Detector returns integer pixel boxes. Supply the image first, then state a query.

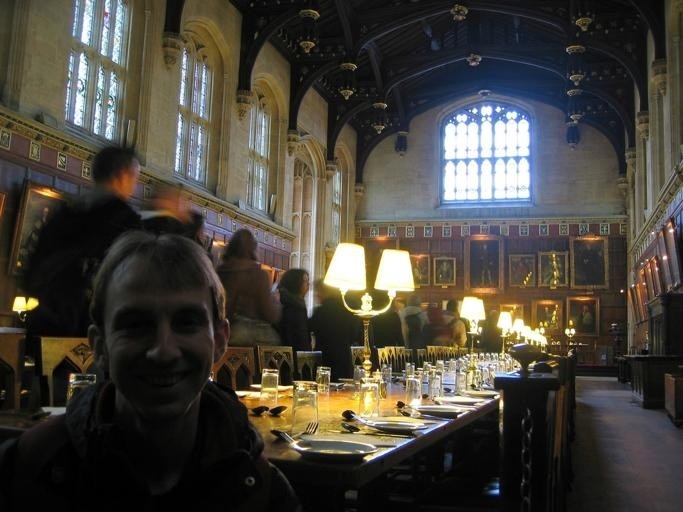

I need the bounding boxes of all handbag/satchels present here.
[227,313,282,347]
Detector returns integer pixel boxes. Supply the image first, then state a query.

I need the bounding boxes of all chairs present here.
[1,325,578,512]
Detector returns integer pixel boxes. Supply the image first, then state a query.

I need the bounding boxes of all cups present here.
[260,352,515,432]
[64,372,97,403]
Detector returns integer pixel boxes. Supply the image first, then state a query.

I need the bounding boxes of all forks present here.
[297,420,320,436]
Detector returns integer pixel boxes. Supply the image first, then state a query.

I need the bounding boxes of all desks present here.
[611,352,682,428]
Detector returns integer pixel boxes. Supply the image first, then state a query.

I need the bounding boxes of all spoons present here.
[0,410,51,420]
[245,404,287,418]
[269,429,289,439]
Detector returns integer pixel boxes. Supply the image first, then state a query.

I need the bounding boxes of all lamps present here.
[296,0,596,159]
[321,241,576,382]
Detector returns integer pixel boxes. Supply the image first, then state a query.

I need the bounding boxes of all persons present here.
[577,241,603,282]
[0,231,304,512]
[512,259,528,284]
[574,302,595,335]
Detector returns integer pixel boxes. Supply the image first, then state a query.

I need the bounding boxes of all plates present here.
[249,383,292,392]
[287,439,378,462]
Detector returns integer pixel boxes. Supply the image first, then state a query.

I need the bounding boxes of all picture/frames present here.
[3,177,80,281]
[408,214,682,340]
